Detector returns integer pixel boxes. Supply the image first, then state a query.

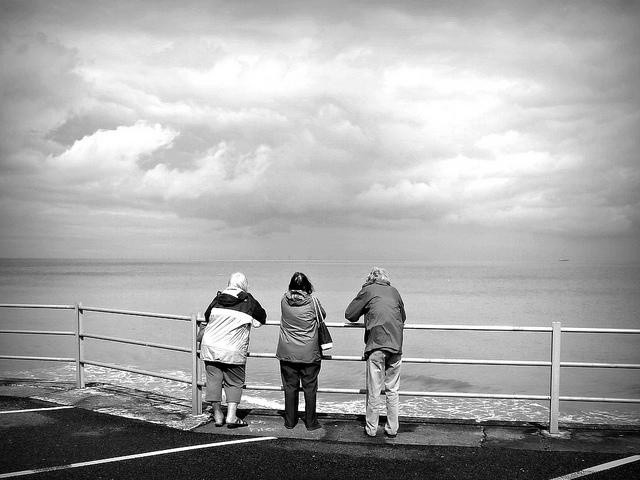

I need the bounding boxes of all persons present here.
[194,273,266,428]
[274,271,326,432]
[343,266,406,437]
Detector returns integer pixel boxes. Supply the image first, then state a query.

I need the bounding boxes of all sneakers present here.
[285,418,297,429]
[306,419,321,431]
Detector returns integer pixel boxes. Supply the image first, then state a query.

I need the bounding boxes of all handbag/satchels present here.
[318,322,333,350]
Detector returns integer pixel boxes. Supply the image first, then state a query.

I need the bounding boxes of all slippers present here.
[215,415,226,427]
[227,417,249,429]
